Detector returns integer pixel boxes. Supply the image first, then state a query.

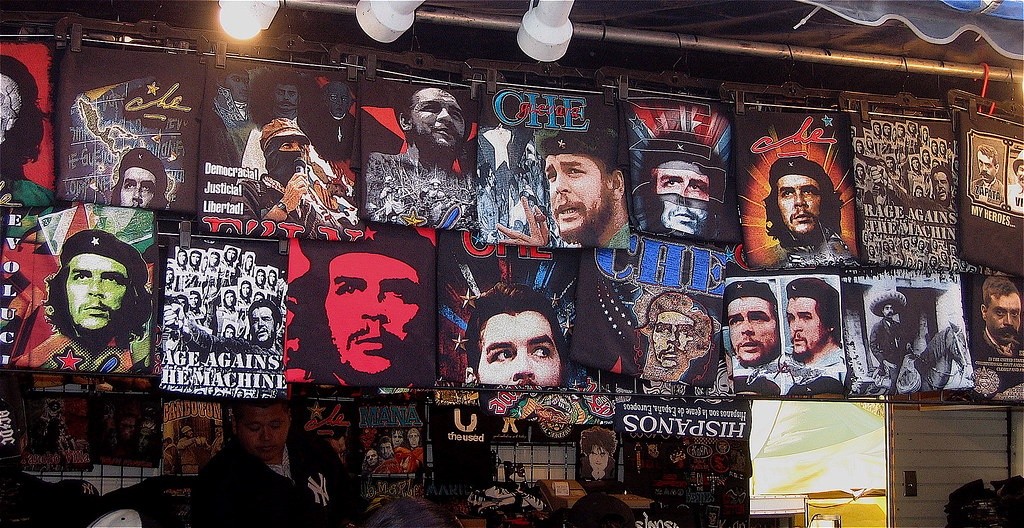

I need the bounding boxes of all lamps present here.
[517,0,574,62]
[356,0,426,42]
[219,0,280,39]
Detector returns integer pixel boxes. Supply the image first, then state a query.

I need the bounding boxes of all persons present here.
[186,397,366,528]
